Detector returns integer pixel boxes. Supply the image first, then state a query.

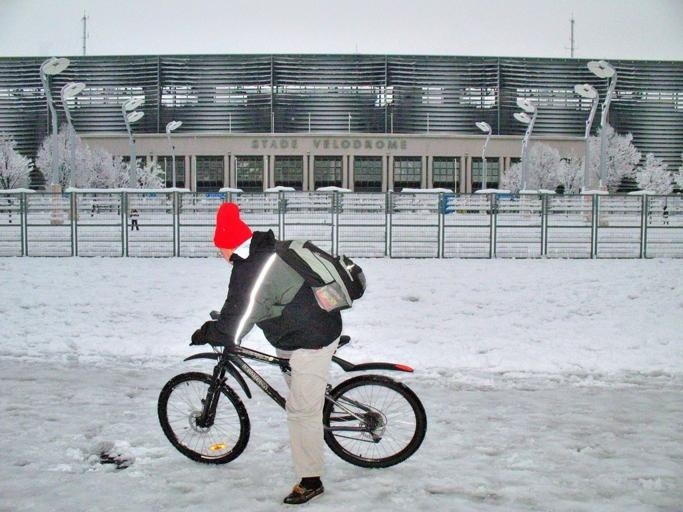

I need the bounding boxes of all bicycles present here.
[157,335,428,469]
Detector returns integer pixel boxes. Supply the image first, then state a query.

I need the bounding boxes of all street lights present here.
[39,55,183,224]
[475,59,618,227]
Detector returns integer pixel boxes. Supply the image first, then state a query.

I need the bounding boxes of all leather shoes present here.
[283,483,325,506]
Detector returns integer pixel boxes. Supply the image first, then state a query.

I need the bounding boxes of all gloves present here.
[191,321,228,345]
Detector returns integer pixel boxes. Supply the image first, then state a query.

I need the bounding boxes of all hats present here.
[214,202,253,249]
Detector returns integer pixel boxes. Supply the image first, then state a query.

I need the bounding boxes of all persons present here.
[196,202,367,504]
[130,209,139,230]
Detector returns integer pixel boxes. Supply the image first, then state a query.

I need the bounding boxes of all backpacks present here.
[254,239,367,314]
